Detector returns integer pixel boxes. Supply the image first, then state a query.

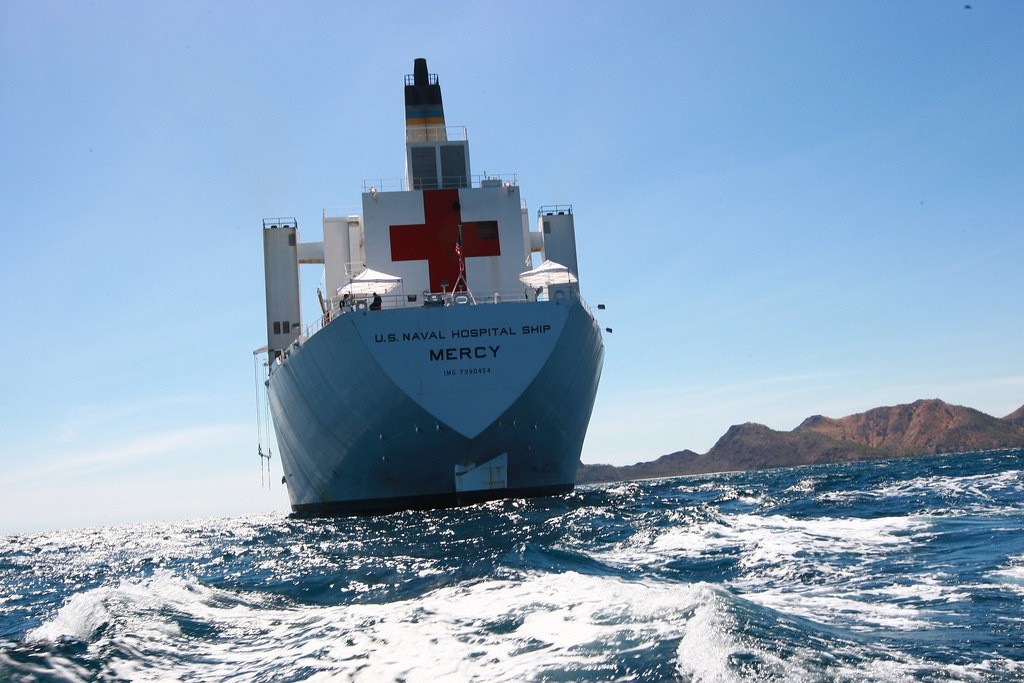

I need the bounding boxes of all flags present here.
[455,233,466,271]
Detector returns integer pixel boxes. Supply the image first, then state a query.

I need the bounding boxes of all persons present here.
[339,294,351,309]
[369,293,382,310]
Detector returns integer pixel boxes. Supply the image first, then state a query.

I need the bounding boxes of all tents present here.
[518,258,578,301]
[337,268,405,314]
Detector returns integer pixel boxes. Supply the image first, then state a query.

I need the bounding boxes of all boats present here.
[261,57,605,514]
